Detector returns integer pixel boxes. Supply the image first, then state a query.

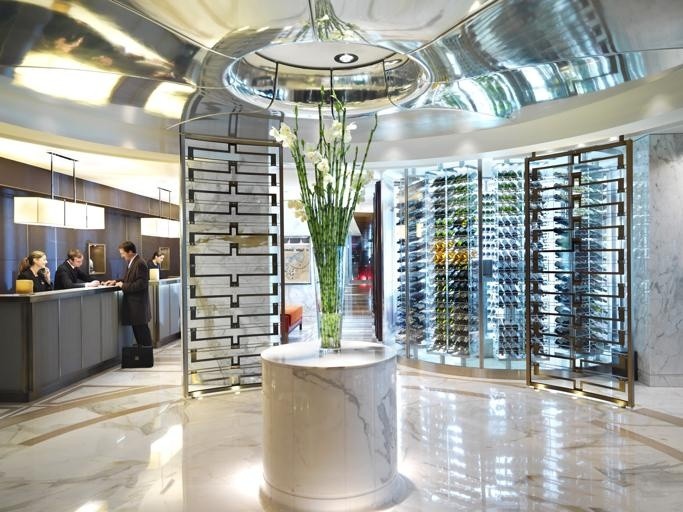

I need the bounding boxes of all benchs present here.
[279,305,303,342]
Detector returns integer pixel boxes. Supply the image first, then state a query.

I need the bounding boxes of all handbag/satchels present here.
[121,342,153,368]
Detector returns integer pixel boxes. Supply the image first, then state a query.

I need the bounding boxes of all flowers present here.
[269,86,378,352]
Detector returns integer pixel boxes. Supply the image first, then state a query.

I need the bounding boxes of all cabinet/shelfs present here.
[527,139,633,409]
[377,157,525,379]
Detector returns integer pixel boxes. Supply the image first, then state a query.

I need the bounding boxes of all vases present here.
[311,245,344,353]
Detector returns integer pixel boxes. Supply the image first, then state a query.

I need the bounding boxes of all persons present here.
[16,251,53,293]
[53,248,104,290]
[102,241,152,346]
[147,251,168,279]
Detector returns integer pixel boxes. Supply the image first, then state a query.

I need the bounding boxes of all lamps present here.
[13,152,105,230]
[140,188,180,239]
